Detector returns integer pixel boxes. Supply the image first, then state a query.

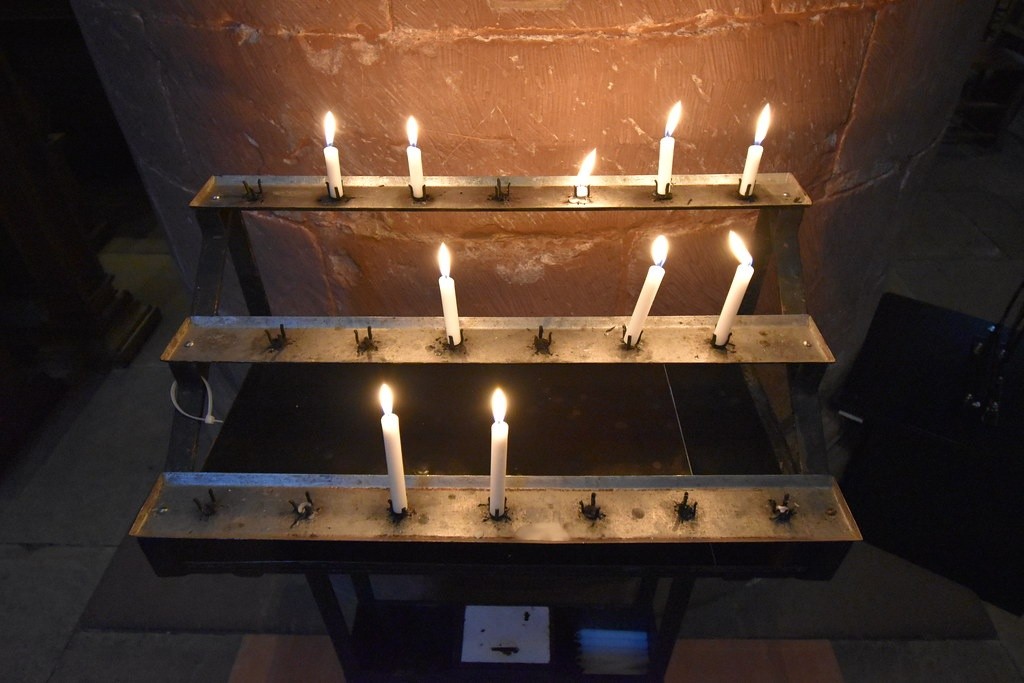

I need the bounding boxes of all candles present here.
[623,235,669,347]
[490,385,510,517]
[437,243,463,346]
[322,111,344,199]
[377,383,410,515]
[739,101,772,197]
[574,147,597,197]
[713,229,754,345]
[657,101,681,194]
[405,116,425,199]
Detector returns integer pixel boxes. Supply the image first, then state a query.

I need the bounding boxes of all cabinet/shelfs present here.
[128,173,865,683]
